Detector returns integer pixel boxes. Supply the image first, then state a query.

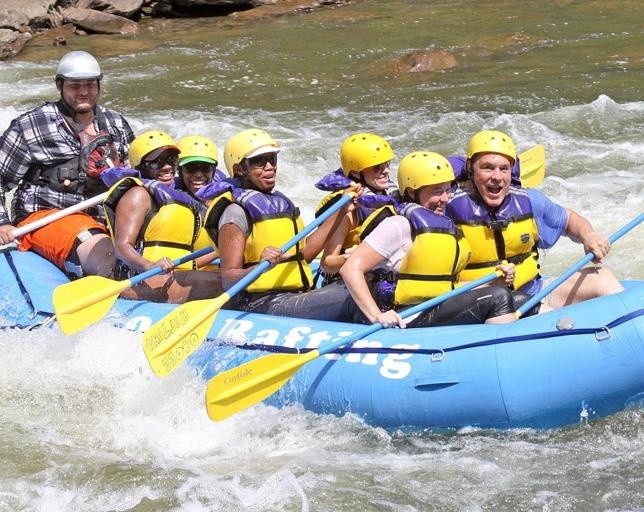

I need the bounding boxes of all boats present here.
[0,224,644,440]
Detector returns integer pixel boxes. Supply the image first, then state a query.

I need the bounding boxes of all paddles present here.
[52,247,214,335]
[518,144,546,187]
[205,259,511,420]
[141,182,357,378]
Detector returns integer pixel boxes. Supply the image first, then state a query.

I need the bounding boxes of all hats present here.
[244,144,282,159]
[179,156,216,166]
[144,146,181,161]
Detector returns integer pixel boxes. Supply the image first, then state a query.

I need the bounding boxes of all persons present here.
[444,130,624,314]
[201,128,377,325]
[101,130,232,309]
[339,151,517,331]
[178,134,226,213]
[0,49,136,279]
[314,133,399,286]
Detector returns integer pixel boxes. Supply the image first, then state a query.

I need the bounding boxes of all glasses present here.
[185,165,213,174]
[142,155,178,171]
[250,156,279,169]
[371,160,391,177]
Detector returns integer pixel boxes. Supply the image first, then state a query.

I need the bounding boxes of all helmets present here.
[129,131,180,169]
[341,133,393,179]
[175,136,218,166]
[54,50,103,81]
[398,152,457,196]
[224,128,277,177]
[465,131,517,167]
